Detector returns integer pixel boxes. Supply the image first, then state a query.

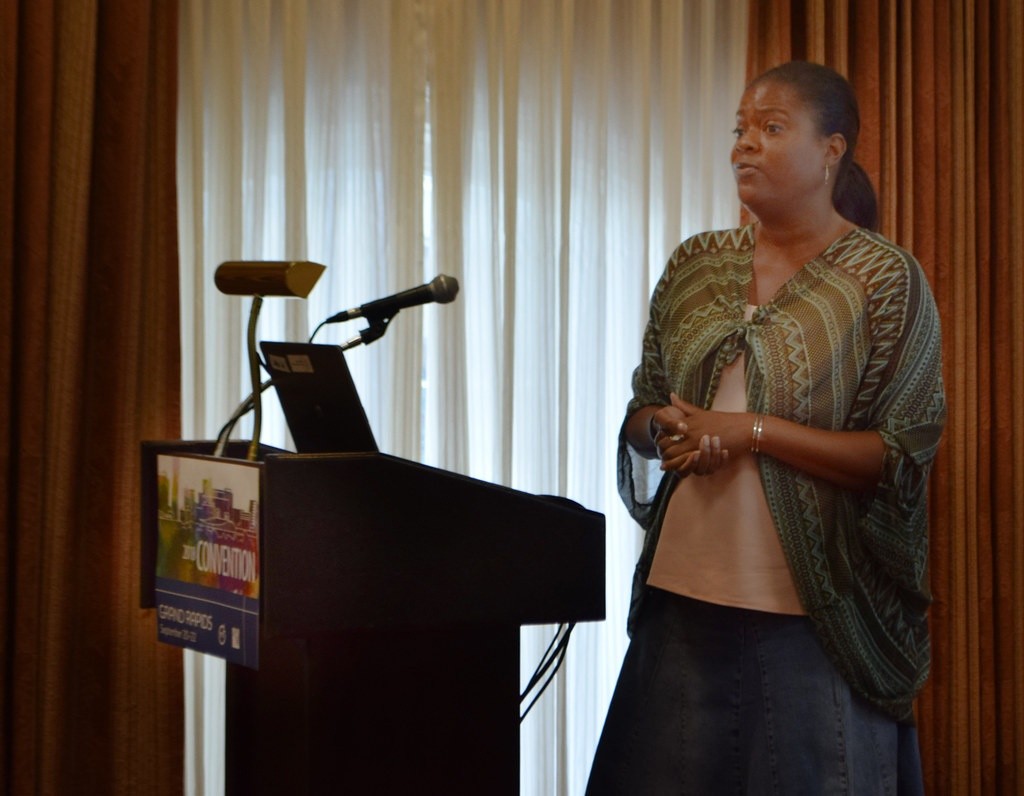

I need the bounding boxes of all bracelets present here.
[756,415,764,452]
[751,415,759,452]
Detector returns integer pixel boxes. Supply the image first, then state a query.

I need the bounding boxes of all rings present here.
[670,432,684,441]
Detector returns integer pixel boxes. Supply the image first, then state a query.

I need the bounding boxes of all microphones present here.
[326,275,459,323]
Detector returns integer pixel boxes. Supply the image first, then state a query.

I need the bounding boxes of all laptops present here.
[259,341,378,454]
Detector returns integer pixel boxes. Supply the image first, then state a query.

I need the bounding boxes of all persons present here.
[584,59,947,796]
